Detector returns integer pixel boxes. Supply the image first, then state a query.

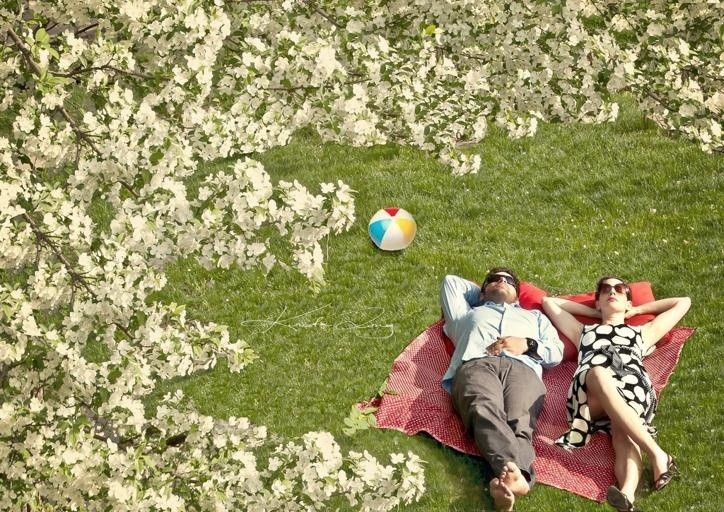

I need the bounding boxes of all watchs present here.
[524,336,538,355]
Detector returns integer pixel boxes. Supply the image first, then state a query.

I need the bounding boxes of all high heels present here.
[653,455,681,491]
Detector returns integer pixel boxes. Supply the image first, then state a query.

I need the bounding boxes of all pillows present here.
[554,283,673,361]
[445,282,546,356]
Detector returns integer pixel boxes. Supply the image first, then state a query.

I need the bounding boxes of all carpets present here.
[362,313,694,505]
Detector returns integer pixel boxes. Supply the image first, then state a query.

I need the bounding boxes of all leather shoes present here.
[606,486,633,512]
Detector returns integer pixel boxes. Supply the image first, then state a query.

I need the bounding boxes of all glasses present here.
[598,283,630,293]
[483,275,515,288]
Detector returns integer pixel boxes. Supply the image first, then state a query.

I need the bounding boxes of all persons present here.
[540,275,692,512]
[439,266,564,512]
